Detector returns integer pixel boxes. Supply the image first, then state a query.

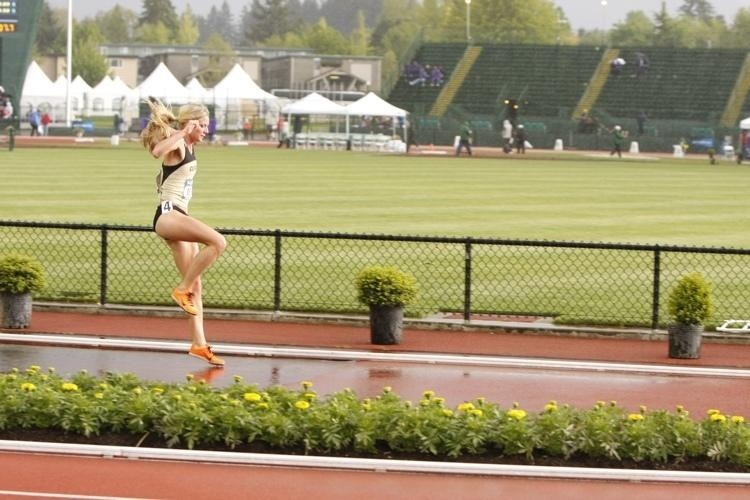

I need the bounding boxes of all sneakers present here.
[189,344,225,365]
[171,288,197,315]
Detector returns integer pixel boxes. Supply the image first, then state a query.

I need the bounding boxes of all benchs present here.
[386,41,749,120]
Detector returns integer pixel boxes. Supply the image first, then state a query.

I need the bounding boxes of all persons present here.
[241,112,298,148]
[404,57,444,89]
[139,96,228,368]
[451,118,526,158]
[678,129,750,168]
[573,52,650,159]
[1,97,54,137]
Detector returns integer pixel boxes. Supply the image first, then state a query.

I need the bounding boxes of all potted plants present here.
[666,267,715,360]
[353,263,419,344]
[0,252,46,332]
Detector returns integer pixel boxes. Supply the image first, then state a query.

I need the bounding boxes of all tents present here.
[279,91,411,150]
[16,59,276,139]
